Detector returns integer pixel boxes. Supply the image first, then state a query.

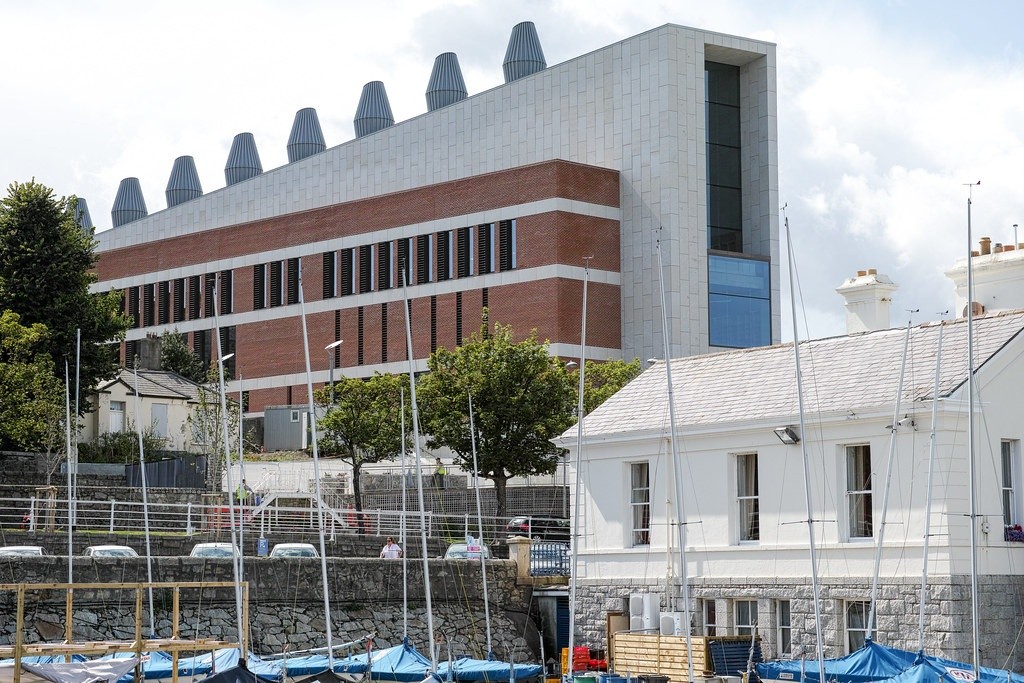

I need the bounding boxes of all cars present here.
[270,542,320,557]
[190,542,240,558]
[0,545,49,557]
[436,541,500,560]
[80,545,139,558]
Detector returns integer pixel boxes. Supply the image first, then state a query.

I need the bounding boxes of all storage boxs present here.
[562,647,607,675]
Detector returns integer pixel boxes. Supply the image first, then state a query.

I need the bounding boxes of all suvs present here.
[506,513,570,546]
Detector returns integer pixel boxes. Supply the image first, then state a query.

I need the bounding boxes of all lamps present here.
[774,426,800,445]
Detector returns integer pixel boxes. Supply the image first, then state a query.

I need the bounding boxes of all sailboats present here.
[0,197,1023,683]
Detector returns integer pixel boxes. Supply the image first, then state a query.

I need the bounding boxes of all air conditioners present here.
[660,611,692,635]
[630,593,660,634]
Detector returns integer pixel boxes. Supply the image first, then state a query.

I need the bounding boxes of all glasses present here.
[386,540,392,542]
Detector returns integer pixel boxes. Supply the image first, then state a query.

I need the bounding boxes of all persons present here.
[237,479,254,520]
[380,537,402,558]
[431,458,445,490]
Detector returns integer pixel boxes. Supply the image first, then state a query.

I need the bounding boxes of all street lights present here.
[324,339,344,434]
